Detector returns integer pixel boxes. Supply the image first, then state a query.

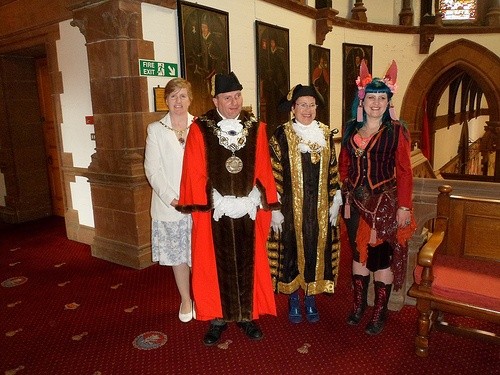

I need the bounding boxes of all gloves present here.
[270,209,284,233]
[328,202,339,226]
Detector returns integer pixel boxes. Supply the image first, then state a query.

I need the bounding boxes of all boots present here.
[364,279,391,335]
[289,290,302,323]
[304,295,319,323]
[348,274,370,325]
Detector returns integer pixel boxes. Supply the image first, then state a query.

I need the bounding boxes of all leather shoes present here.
[205,321,228,343]
[237,320,262,339]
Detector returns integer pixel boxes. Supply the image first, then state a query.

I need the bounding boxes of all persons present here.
[179,71,280,345]
[265,83,344,324]
[143,78,199,322]
[338,59,417,335]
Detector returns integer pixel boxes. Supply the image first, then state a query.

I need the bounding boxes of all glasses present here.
[294,102,319,109]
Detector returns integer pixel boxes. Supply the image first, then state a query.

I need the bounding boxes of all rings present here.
[405,220,411,225]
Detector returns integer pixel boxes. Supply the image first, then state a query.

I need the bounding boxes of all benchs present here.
[407,184,500,358]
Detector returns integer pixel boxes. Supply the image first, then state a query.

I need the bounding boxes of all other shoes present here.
[193,301,197,319]
[178,299,192,322]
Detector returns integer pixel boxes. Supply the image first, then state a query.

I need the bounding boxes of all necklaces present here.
[172,129,188,143]
[354,127,374,157]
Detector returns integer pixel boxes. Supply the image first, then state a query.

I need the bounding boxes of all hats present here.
[278,84,325,112]
[210,72,243,97]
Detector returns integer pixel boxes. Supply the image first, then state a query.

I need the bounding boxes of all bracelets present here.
[399,207,410,211]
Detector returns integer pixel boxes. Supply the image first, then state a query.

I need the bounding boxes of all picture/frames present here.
[177,0,373,148]
